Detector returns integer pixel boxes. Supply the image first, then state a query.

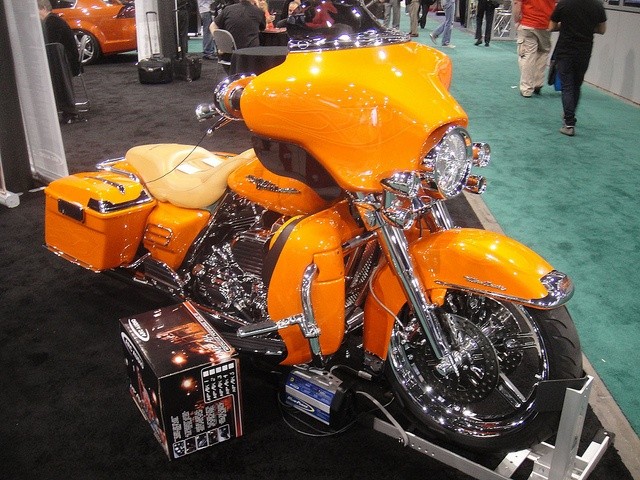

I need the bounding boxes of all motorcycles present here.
[40,0,583,457]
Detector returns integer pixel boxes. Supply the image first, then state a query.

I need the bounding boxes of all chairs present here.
[72,34,89,116]
[213,29,236,85]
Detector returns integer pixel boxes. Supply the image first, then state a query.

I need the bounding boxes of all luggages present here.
[171,10,202,82]
[138,11,169,84]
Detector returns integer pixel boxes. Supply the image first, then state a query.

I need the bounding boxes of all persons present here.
[274,0,302,29]
[255,0,274,29]
[429,0,457,47]
[511,0,562,98]
[548,0,608,136]
[378,0,402,30]
[36,0,79,125]
[404,0,421,38]
[474,0,499,47]
[197,0,220,61]
[459,0,468,28]
[208,0,265,76]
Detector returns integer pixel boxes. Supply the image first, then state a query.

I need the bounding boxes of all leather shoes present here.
[203,54,218,60]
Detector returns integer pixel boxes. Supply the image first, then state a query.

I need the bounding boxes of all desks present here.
[496,12,513,37]
[230,46,287,74]
[258,29,289,40]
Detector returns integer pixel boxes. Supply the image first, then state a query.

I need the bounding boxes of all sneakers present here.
[474,39,482,45]
[442,43,456,48]
[561,113,566,124]
[429,33,437,45]
[408,33,419,37]
[560,126,574,136]
[520,90,531,97]
[533,86,544,94]
[485,42,490,47]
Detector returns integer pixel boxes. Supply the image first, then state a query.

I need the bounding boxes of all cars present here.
[37,0,137,67]
